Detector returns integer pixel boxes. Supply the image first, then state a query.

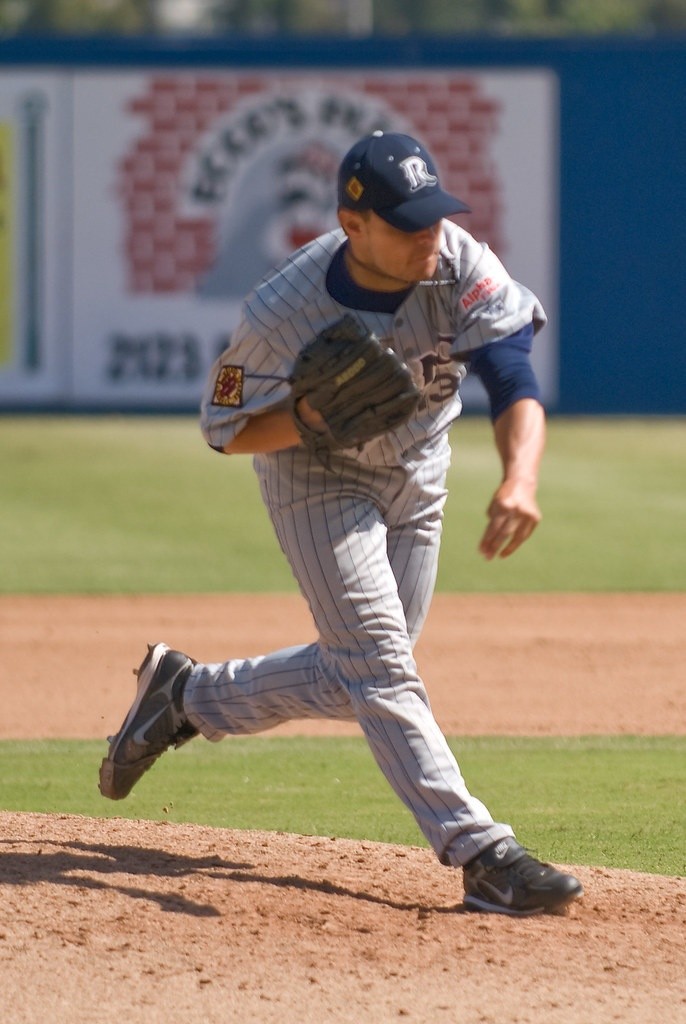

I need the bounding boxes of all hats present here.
[337,132,474,231]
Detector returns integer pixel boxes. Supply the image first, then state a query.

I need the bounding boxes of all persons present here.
[97,128,585,919]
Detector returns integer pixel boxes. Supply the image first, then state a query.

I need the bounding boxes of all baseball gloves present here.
[261,309,426,476]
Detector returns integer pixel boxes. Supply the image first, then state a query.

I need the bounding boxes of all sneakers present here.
[98,642,202,800]
[463,835,584,914]
